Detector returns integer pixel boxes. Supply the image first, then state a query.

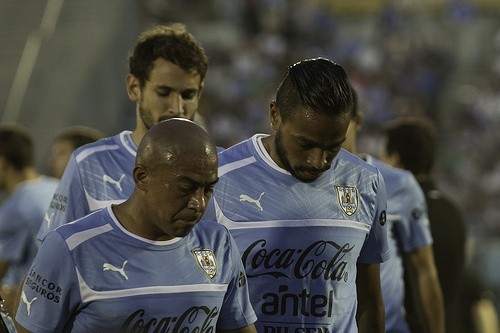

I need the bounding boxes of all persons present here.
[13,118,259,333]
[36,22,228,245]
[201,57,392,333]
[0,87,500,333]
